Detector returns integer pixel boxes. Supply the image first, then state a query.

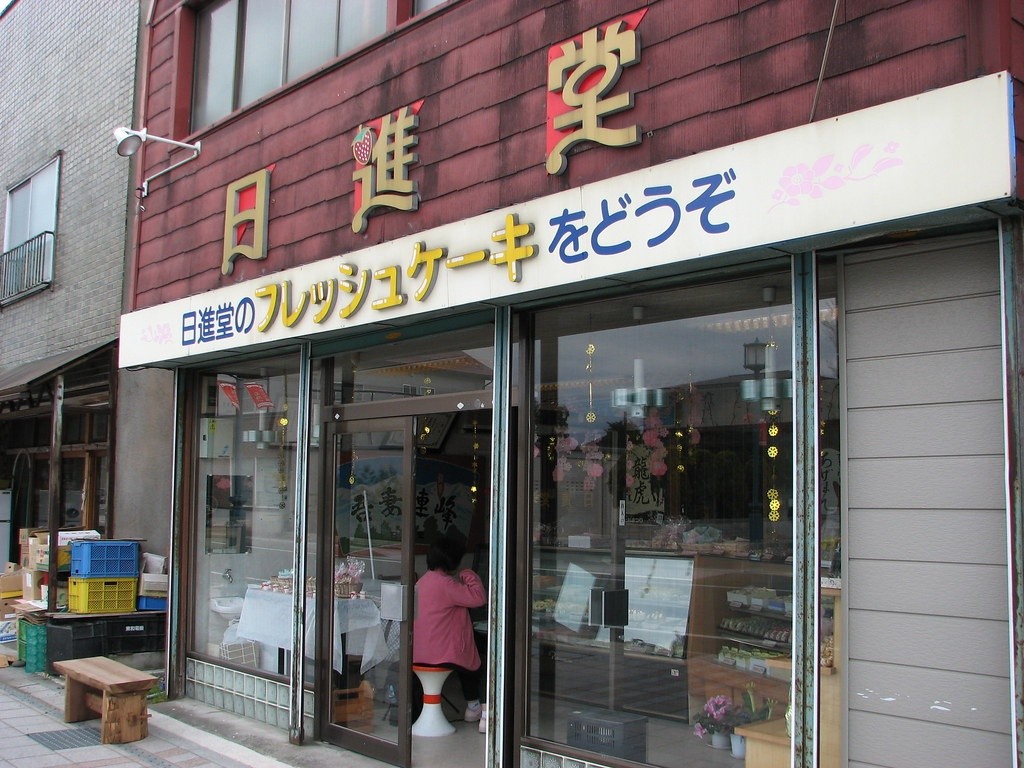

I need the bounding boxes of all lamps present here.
[610,306,670,419]
[742,287,792,411]
[242,367,280,449]
[112,126,202,157]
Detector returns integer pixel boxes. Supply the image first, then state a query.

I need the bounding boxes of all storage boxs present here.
[0,529,168,664]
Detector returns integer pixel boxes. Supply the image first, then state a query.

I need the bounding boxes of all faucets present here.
[223,568,233,583]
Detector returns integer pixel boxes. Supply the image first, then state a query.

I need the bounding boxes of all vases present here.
[711,730,747,757]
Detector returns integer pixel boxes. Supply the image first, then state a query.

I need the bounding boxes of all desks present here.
[246,579,459,721]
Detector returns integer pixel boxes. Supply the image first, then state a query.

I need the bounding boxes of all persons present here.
[413,537,490,733]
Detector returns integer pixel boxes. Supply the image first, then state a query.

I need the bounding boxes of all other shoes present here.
[465,706,481,722]
[479,718,486,732]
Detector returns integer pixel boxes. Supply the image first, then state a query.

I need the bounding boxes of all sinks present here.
[210,597,244,619]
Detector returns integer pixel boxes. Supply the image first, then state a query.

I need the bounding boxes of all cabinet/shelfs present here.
[529,547,695,726]
[694,554,842,768]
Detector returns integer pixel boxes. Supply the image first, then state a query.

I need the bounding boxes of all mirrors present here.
[205,474,254,555]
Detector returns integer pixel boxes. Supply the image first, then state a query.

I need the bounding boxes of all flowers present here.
[692,682,778,739]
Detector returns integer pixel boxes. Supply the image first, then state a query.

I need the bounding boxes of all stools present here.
[412,665,456,737]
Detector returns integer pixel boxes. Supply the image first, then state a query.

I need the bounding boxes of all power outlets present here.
[142,181,149,198]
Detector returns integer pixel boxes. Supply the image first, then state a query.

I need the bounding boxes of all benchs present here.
[52,656,158,744]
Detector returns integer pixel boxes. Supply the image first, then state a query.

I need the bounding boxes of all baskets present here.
[678,540,789,563]
[305,576,316,591]
[334,582,363,598]
[270,576,293,589]
